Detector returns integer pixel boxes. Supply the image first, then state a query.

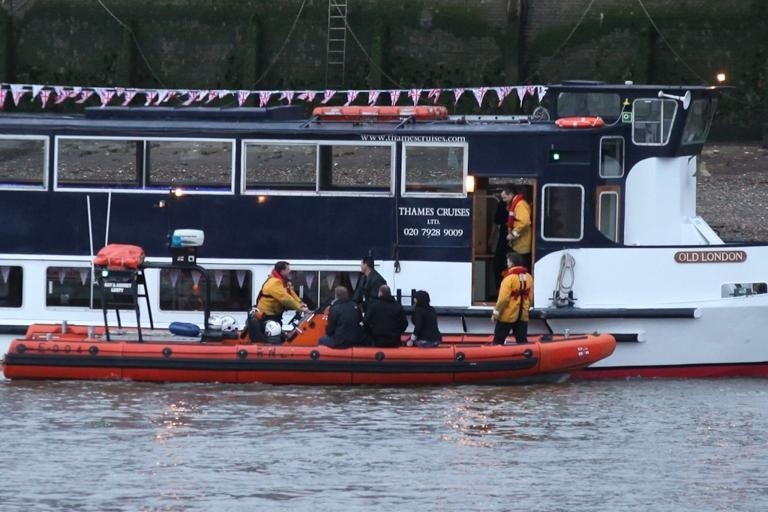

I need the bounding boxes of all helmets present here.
[220,317,240,332]
[208,316,222,332]
[266,321,283,338]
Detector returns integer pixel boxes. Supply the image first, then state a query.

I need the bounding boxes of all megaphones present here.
[658,88,691,110]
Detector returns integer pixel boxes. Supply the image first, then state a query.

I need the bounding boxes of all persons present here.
[352,256,388,314]
[317,285,363,350]
[490,250,534,348]
[364,284,409,347]
[493,182,518,301]
[500,184,532,274]
[406,290,443,349]
[255,260,309,327]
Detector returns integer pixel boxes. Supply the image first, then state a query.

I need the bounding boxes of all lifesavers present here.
[554,115,605,128]
[170,322,201,337]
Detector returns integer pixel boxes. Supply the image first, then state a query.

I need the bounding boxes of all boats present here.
[1,83,767,381]
[3,325,617,382]
